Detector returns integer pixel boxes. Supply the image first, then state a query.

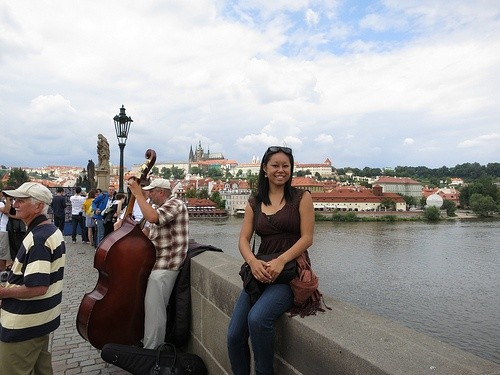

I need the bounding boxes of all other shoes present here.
[5,263,13,271]
[82,241,85,243]
[1,273,8,282]
[90,241,95,246]
[72,241,75,243]
[64,241,67,244]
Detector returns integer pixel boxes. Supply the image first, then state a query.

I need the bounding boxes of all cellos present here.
[76,149,156,350]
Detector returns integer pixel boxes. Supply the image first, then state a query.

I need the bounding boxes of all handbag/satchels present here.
[238,251,297,298]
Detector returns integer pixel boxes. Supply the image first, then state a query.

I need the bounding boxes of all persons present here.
[0,186,27,272]
[226,146,316,375]
[0,180,66,375]
[51,187,66,233]
[69,176,150,251]
[126,177,190,351]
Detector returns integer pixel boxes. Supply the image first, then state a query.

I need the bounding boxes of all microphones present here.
[0,272,8,288]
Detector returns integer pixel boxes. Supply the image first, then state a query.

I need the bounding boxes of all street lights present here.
[113,105,134,194]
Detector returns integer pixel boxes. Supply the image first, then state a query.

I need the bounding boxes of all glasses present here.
[266,146,292,155]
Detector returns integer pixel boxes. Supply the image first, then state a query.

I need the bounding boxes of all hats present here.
[142,178,171,191]
[2,182,54,206]
